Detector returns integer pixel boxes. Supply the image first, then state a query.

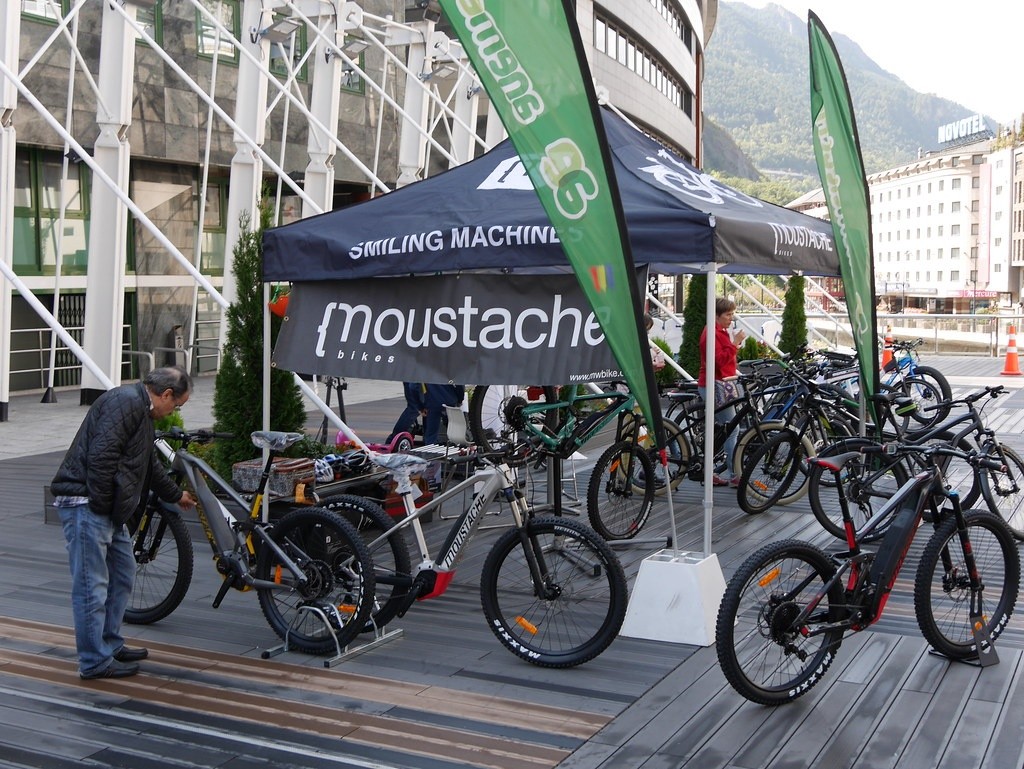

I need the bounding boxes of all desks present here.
[220,466,423,521]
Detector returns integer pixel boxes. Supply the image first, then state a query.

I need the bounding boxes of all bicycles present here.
[123,337,1024,708]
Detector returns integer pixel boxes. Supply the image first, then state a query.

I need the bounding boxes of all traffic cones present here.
[878,324,898,374]
[999,325,1022,375]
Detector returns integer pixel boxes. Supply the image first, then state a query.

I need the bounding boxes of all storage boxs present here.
[383,492,435,524]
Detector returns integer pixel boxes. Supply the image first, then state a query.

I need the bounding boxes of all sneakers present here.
[113,645,148,662]
[729,475,754,488]
[701,473,729,487]
[80,658,139,679]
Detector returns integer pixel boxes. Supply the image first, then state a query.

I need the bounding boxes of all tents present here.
[252,89,867,560]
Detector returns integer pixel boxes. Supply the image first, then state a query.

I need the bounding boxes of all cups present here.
[655,352,664,365]
[733,329,741,345]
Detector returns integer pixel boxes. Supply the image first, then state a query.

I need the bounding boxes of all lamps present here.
[325,40,372,64]
[250,18,302,43]
[418,65,457,86]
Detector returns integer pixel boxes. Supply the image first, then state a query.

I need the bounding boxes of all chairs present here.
[525,454,578,507]
[439,403,524,531]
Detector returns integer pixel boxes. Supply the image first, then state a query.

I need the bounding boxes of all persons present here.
[698,297,746,488]
[47,364,196,679]
[641,311,666,372]
[385,383,464,443]
